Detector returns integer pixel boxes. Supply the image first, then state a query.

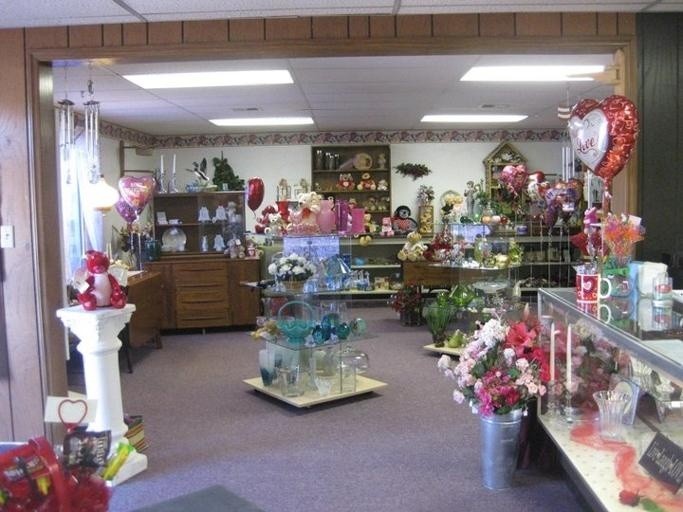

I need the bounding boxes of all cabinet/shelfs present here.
[404,261,504,324]
[256,237,404,308]
[244,231,389,411]
[120,272,162,372]
[311,142,392,233]
[149,191,247,258]
[483,141,529,202]
[487,233,578,293]
[140,258,260,336]
[537,288,683,511]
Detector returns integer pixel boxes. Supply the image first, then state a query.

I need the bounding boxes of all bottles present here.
[314,149,340,170]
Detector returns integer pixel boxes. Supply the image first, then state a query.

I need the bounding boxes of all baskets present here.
[276,301,316,345]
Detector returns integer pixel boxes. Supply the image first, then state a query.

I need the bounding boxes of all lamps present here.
[87,173,119,217]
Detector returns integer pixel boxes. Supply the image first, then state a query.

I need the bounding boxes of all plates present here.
[352,153,373,171]
[161,228,187,246]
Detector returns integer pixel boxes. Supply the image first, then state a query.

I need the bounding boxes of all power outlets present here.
[1,227,15,249]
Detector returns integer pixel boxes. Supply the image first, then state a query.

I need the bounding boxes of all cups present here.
[576,301,612,323]
[651,278,675,308]
[652,307,672,331]
[223,183,228,191]
[247,248,258,257]
[577,274,612,303]
[592,390,631,437]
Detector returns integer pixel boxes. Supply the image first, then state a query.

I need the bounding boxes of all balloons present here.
[501,163,584,232]
[244,176,264,210]
[114,196,144,225]
[569,95,640,181]
[118,174,157,215]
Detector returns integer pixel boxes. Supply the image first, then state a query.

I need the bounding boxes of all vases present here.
[282,281,306,291]
[480,414,520,491]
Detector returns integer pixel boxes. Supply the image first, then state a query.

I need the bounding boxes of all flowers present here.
[437,319,550,417]
[553,321,615,412]
[268,253,317,281]
[441,192,463,220]
[392,286,421,315]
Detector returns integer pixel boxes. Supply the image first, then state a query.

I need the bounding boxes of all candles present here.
[160,154,164,173]
[172,154,177,172]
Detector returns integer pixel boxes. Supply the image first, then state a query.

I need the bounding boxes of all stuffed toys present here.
[254,171,429,261]
